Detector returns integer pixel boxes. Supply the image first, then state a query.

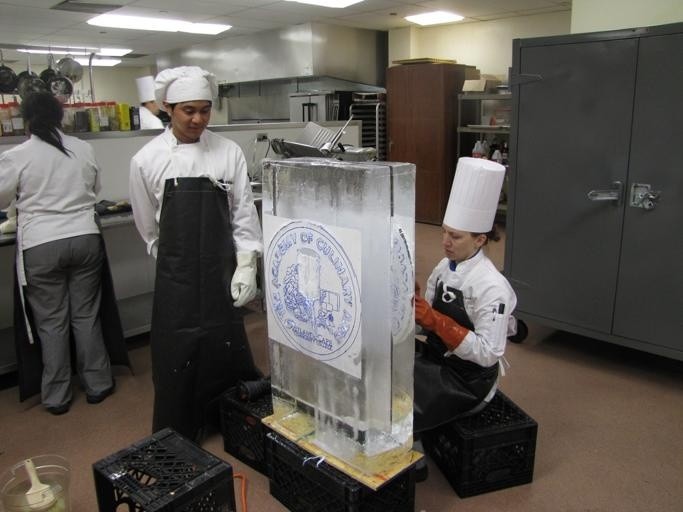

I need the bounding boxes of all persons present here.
[136,76,164,130]
[414,157,517,485]
[129,65,263,445]
[0,91,135,415]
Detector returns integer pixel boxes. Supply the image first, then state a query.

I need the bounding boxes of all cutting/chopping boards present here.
[393,59,457,65]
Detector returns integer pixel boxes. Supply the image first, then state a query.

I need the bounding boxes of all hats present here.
[154,66,218,116]
[136,76,156,103]
[443,157,505,233]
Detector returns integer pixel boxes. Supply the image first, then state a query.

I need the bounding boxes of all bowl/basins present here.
[329,151,378,163]
[2,455,73,507]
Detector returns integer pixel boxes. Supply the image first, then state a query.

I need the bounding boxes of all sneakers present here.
[415,465,428,482]
[86,376,115,404]
[48,402,69,414]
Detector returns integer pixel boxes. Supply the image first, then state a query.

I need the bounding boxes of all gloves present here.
[415,281,420,297]
[231,252,257,308]
[151,239,160,259]
[415,294,469,352]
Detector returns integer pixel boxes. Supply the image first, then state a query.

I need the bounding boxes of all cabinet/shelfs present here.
[502,23,683,359]
[457,94,511,224]
[387,64,476,226]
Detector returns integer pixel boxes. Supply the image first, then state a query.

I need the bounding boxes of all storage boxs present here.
[92,426,238,512]
[267,430,417,512]
[420,387,540,500]
[221,382,274,477]
[462,80,502,96]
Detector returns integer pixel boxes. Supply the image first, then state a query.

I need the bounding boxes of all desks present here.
[0,182,263,380]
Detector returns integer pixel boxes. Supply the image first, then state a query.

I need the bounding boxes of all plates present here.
[467,126,509,129]
